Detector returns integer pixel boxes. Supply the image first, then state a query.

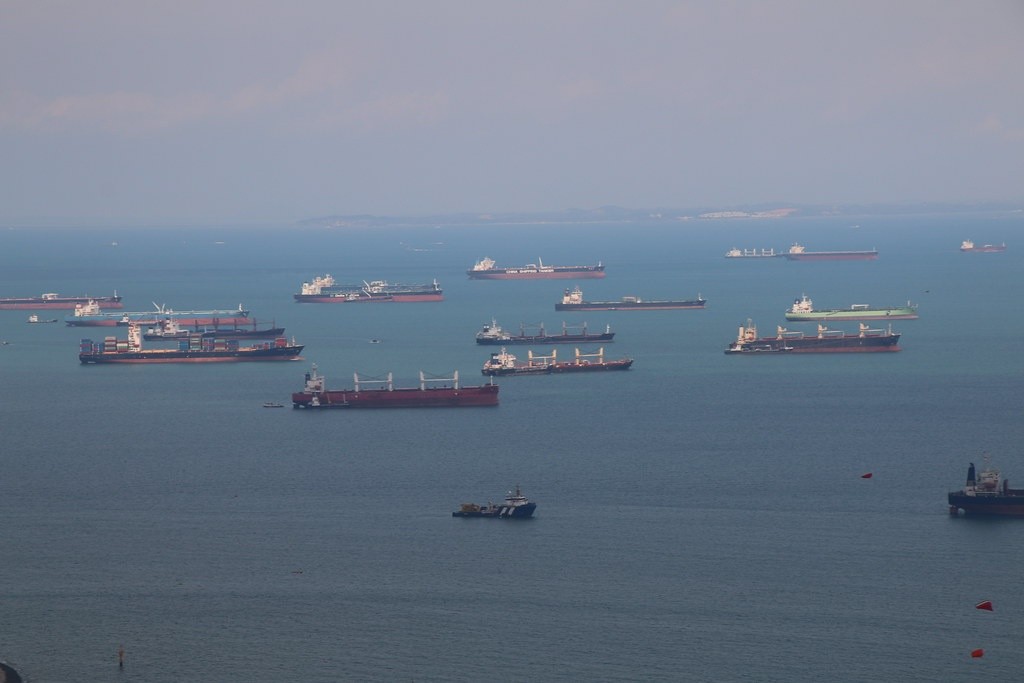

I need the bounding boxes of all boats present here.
[452,484,536,518]
[481,346,634,376]
[64,298,251,328]
[291,363,499,407]
[475,317,615,346]
[785,293,919,320]
[960,238,1008,252]
[947,448,1024,517]
[312,273,440,290]
[143,317,286,342]
[554,285,709,310]
[724,319,902,353]
[725,246,784,258]
[27,314,57,324]
[0,290,124,310]
[464,256,606,280]
[263,401,283,408]
[781,243,879,260]
[79,323,306,362]
[293,281,444,303]
